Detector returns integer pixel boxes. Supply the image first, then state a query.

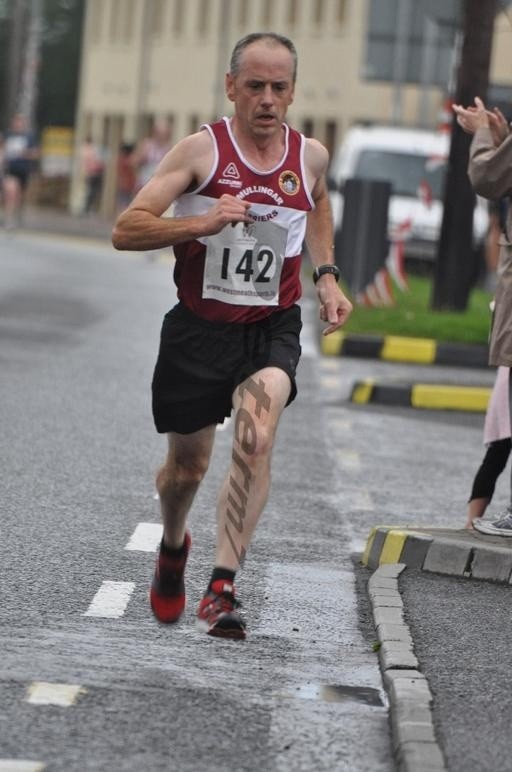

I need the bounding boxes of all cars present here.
[325,119,492,288]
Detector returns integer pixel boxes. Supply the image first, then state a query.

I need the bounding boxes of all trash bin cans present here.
[344,179,388,295]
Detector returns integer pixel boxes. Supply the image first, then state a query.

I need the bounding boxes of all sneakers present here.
[198,579,248,641]
[148,526,191,619]
[471,509,512,536]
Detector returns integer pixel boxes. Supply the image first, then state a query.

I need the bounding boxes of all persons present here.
[80,136,104,213]
[0,114,40,231]
[111,31,353,639]
[462,365,512,530]
[128,117,172,196]
[450,95,512,536]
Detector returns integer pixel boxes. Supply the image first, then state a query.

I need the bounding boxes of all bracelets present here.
[313,264,340,286]
